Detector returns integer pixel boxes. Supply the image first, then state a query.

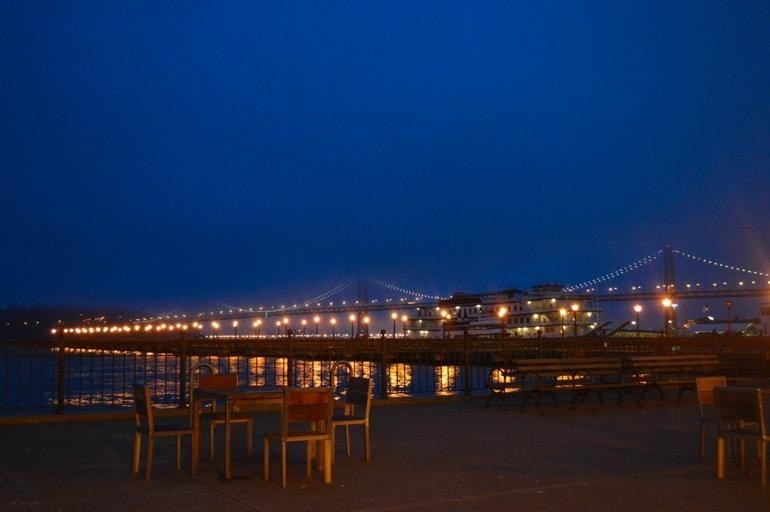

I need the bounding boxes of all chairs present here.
[130,359,375,486]
[692,372,770,486]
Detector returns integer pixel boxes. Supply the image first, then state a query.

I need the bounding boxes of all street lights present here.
[724,300,732,336]
[634,305,642,338]
[440,310,451,339]
[571,303,580,338]
[663,299,670,334]
[498,306,507,337]
[559,308,566,338]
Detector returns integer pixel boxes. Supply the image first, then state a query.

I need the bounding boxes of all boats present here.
[404,284,606,337]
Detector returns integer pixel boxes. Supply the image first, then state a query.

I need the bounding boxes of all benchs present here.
[485,350,730,412]
